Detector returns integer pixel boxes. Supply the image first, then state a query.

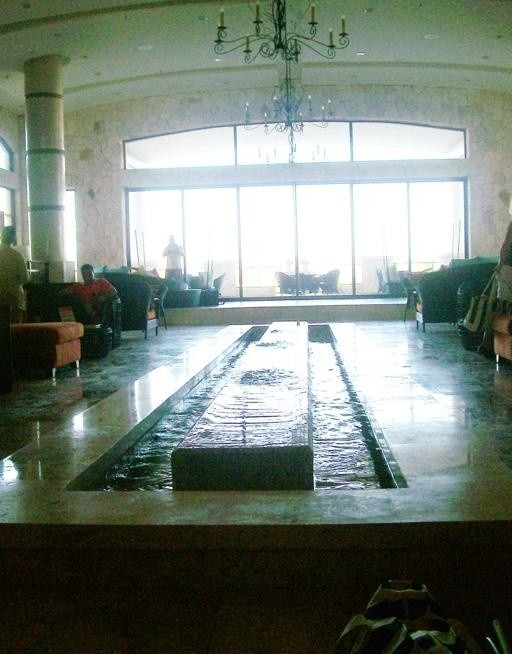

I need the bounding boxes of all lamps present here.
[209,0,351,63]
[238,66,338,134]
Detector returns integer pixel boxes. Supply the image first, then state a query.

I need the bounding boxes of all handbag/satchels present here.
[462,270,497,335]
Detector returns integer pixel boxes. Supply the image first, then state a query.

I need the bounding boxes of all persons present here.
[0,226,29,323]
[478,221,512,360]
[65,264,117,323]
[163,235,184,279]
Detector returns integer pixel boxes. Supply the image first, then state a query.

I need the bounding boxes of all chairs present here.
[375,249,512,374]
[274,267,340,297]
[0,265,227,388]
